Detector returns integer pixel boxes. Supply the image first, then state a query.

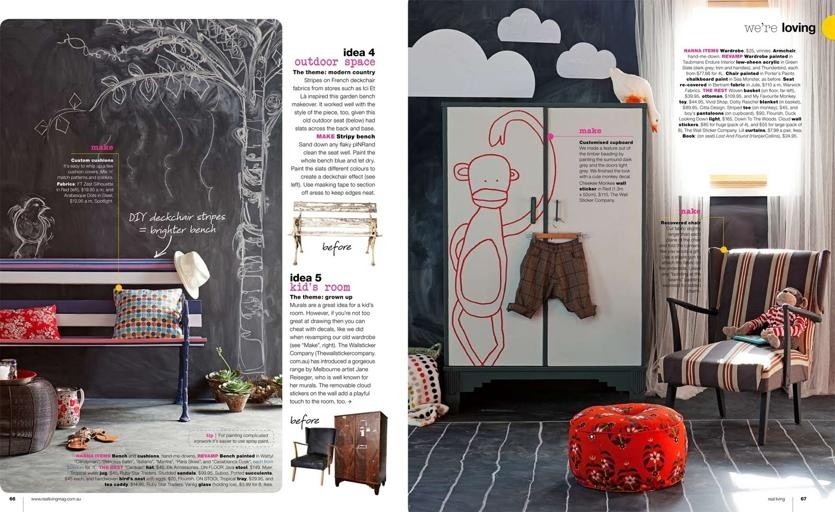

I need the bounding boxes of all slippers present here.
[91,428,118,443]
[67,427,91,451]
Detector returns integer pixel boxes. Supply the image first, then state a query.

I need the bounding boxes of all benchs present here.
[289,216,383,265]
[0,254,208,423]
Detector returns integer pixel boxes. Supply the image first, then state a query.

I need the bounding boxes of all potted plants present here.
[201,368,252,412]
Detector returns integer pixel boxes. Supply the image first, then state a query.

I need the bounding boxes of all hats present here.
[174,250,211,300]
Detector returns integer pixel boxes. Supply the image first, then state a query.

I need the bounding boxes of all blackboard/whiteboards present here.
[2,0,644,400]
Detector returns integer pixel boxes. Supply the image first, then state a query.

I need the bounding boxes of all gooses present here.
[610,67,661,133]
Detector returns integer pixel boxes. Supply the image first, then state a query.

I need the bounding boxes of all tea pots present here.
[56,386,85,428]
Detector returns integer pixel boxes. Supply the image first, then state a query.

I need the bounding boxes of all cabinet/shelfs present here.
[334,410,389,495]
[438,98,653,413]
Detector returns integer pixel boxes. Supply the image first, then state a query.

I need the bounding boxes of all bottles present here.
[0,359,17,380]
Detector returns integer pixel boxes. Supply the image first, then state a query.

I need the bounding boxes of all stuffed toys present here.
[722,288,808,351]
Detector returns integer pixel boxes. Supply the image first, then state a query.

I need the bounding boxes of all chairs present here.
[661,246,829,447]
[290,426,336,485]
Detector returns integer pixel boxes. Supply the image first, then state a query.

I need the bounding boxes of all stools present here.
[570,402,686,492]
[0,376,57,457]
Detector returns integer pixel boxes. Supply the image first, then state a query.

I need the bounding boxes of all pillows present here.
[0,302,59,343]
[111,287,183,338]
